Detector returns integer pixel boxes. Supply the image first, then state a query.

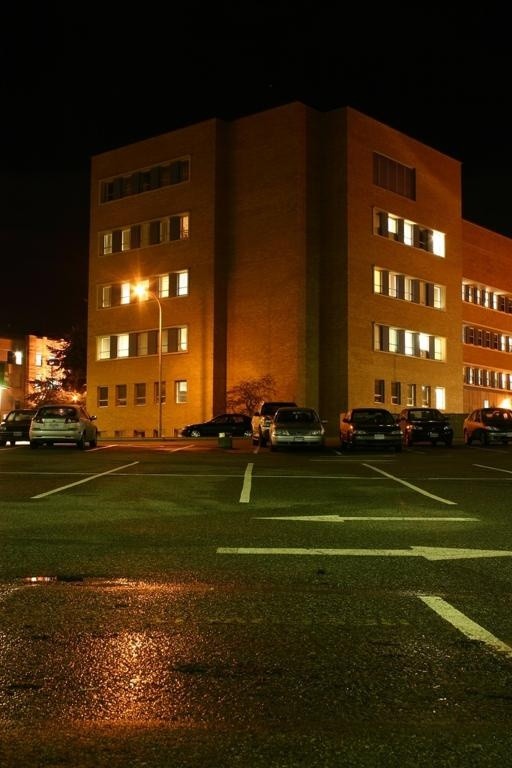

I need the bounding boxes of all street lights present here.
[133,284,162,438]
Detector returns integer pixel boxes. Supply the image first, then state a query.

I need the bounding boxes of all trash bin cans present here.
[219,431,233,448]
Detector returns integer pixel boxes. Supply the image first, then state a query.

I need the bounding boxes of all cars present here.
[0,409,53,447]
[339,408,404,451]
[29,404,97,449]
[182,414,252,438]
[400,408,453,448]
[464,408,512,448]
[251,401,329,446]
